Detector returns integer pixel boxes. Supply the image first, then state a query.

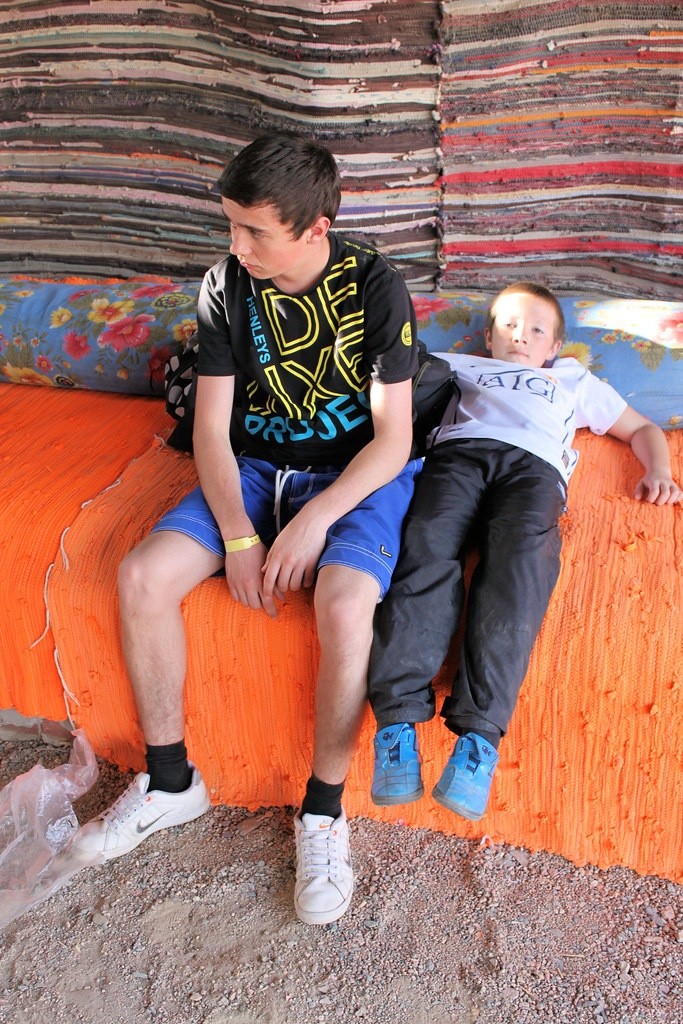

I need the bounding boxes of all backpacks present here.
[406,339,463,463]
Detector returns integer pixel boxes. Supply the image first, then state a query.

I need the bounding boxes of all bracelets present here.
[222,532,262,554]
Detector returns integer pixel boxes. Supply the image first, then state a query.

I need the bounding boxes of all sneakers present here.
[431,732,499,821]
[70,761,210,864]
[293,803,354,925]
[371,722,424,806]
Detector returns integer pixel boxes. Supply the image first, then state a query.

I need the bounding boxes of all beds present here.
[1,274,683,881]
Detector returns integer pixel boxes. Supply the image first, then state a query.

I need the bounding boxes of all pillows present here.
[0,275,204,399]
[409,289,683,431]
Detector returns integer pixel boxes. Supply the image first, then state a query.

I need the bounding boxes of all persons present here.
[365,280,681,822]
[54,128,430,927]
